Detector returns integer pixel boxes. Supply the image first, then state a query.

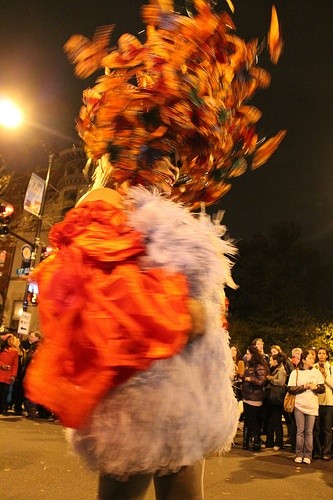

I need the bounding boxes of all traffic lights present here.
[1,200,14,237]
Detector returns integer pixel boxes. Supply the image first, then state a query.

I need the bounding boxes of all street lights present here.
[1,97,57,313]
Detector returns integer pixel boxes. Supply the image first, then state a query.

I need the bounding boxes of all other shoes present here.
[48,418,54,421]
[55,419,60,423]
[294,457,302,463]
[313,453,323,458]
[302,457,310,464]
[25,415,32,419]
[2,410,8,416]
[272,446,280,451]
[15,412,24,416]
[323,454,331,460]
[261,444,266,448]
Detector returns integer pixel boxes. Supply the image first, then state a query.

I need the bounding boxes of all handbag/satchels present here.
[284,391,295,412]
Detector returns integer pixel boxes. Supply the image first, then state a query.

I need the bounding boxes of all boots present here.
[252,427,261,451]
[242,427,250,450]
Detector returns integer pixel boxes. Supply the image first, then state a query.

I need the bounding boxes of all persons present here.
[230,337,333,464]
[0,326,58,422]
[25,140,241,500]
